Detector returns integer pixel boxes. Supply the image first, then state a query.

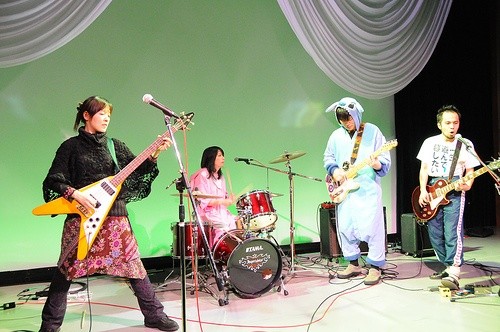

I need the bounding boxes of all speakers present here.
[401,212,437,256]
[319,207,389,258]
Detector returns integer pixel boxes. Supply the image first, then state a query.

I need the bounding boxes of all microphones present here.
[455,134,470,147]
[142,94,180,119]
[235,157,254,162]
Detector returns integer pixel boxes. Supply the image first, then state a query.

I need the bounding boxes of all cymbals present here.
[268,151,306,165]
[269,192,285,197]
[170,191,223,199]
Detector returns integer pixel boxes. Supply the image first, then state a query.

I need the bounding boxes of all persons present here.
[189,146,239,239]
[323,98,391,285]
[38,95,179,332]
[416,106,479,289]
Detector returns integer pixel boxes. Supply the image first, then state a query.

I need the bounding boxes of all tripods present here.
[153,196,225,307]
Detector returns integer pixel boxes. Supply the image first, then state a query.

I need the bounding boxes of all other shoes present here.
[442,277,460,290]
[145,315,179,331]
[364,269,381,284]
[431,266,449,279]
[337,264,362,278]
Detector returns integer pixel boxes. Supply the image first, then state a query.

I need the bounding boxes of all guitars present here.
[31,112,194,260]
[325,138,399,204]
[411,157,500,221]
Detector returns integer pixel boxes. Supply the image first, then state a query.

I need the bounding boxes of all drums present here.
[172,188,282,300]
